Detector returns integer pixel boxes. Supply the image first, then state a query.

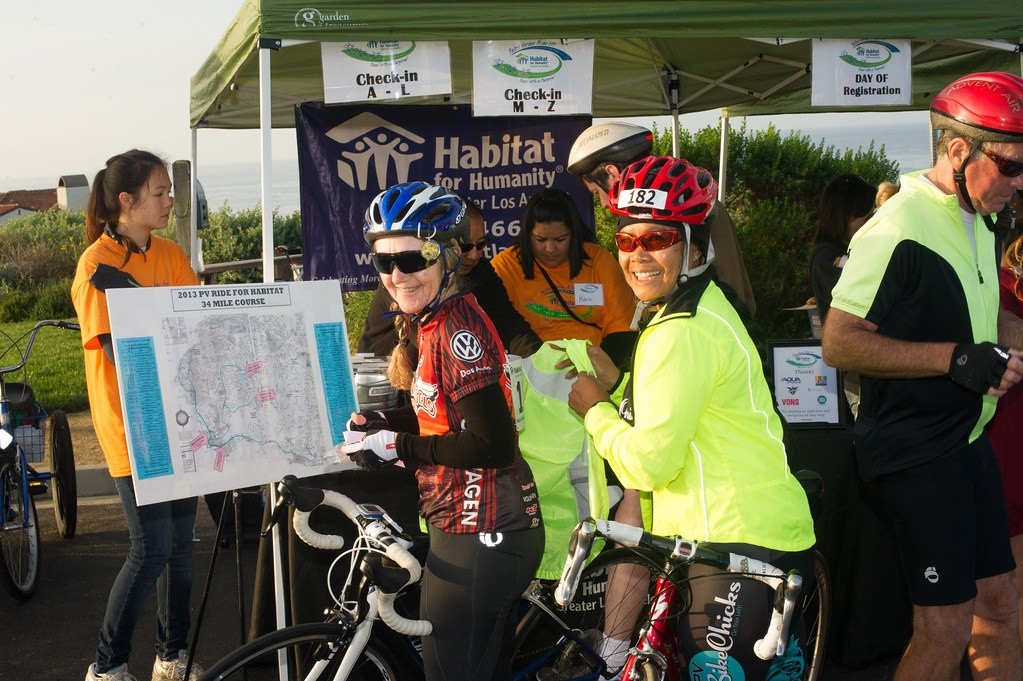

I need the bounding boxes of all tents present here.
[187,0,1023,680]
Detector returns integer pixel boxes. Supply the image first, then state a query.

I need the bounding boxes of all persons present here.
[343,180,545,681]
[548,156,817,681]
[809,174,877,422]
[821,70,1023,681]
[568,123,758,319]
[357,188,543,358]
[489,188,637,345]
[70,149,203,681]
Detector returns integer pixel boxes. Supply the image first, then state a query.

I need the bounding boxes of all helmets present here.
[363,182,470,246]
[567,123,654,175]
[611,156,718,224]
[932,72,1023,142]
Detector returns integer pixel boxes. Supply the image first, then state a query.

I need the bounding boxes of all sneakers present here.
[567,663,627,681]
[84,662,133,681]
[151,648,207,681]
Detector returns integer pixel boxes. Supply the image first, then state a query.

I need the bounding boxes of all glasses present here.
[460,235,490,253]
[370,250,437,275]
[615,228,682,253]
[977,145,1023,178]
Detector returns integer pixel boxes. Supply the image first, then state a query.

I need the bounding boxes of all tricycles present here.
[0,318,82,601]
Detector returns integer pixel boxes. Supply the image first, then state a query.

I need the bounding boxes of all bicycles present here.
[196,473,833,681]
